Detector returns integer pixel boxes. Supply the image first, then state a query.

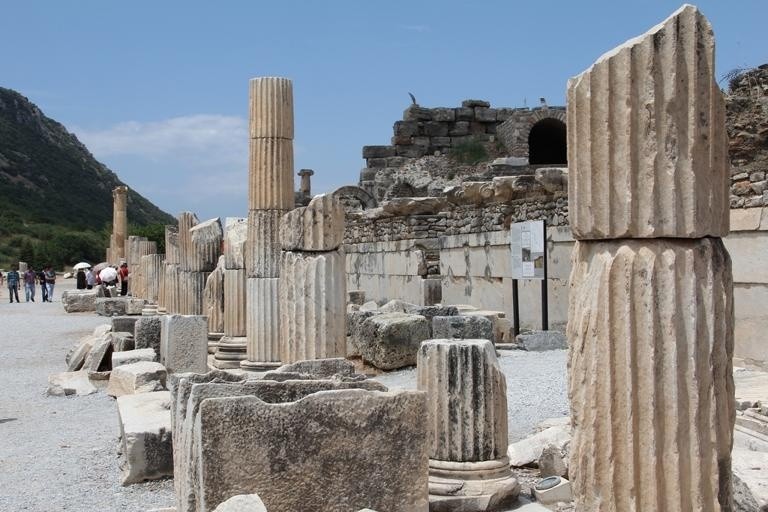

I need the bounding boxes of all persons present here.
[6,264,20,303]
[45,265,56,302]
[72,262,128,296]
[38,265,48,302]
[23,265,38,302]
[0,272,3,285]
